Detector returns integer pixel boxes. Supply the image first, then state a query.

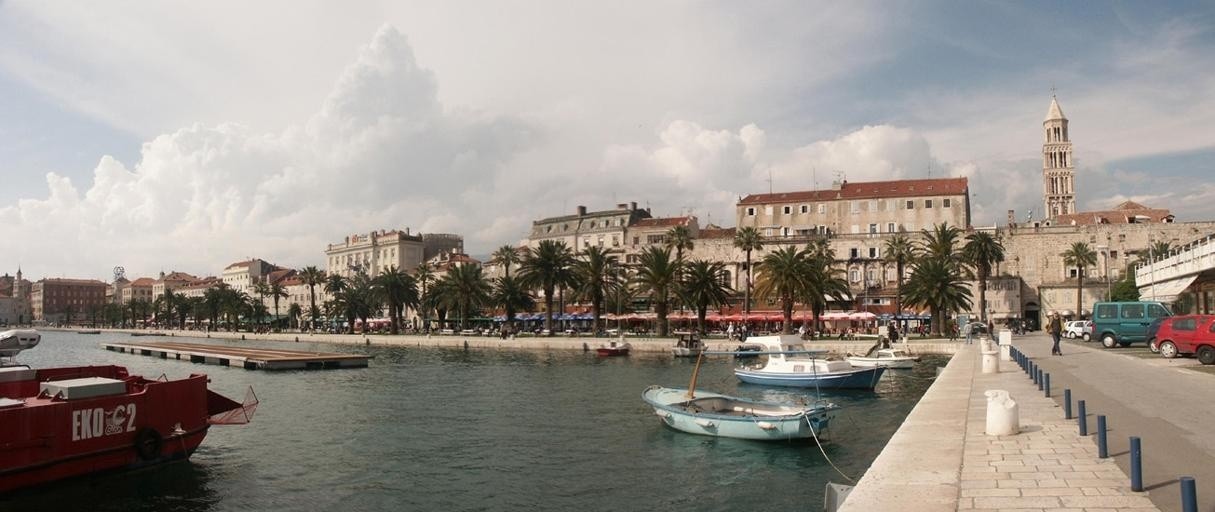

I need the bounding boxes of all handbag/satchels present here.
[1045,324,1053,334]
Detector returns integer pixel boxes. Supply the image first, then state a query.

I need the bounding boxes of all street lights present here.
[1098,245,1112,301]
[1135,215,1154,302]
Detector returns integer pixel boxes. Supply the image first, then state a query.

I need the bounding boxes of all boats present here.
[596,342,633,358]
[642,384,843,442]
[0,362,259,490]
[0,329,42,358]
[844,349,919,369]
[672,332,708,357]
[734,335,887,391]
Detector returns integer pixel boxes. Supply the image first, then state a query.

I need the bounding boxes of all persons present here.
[987,319,996,341]
[1001,316,1036,336]
[1047,311,1065,355]
[944,319,960,341]
[963,320,973,344]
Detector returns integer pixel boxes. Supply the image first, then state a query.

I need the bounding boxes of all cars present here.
[970,322,988,333]
[1146,314,1215,364]
[1061,320,1092,342]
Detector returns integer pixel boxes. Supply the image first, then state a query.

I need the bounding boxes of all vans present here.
[1092,302,1173,353]
